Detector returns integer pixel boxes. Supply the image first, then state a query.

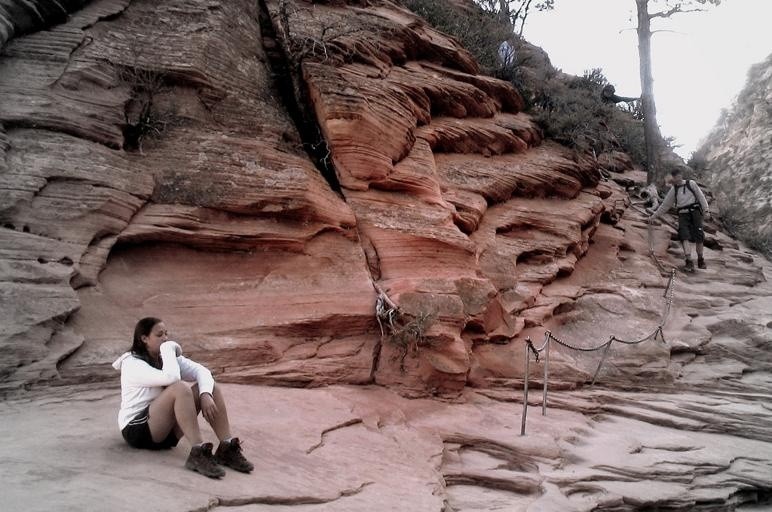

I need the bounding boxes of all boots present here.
[186,437,253,478]
[698,258,706,269]
[683,259,694,272]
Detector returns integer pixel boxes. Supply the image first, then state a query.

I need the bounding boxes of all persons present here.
[498,38,516,66]
[648,169,711,273]
[112,317,254,477]
